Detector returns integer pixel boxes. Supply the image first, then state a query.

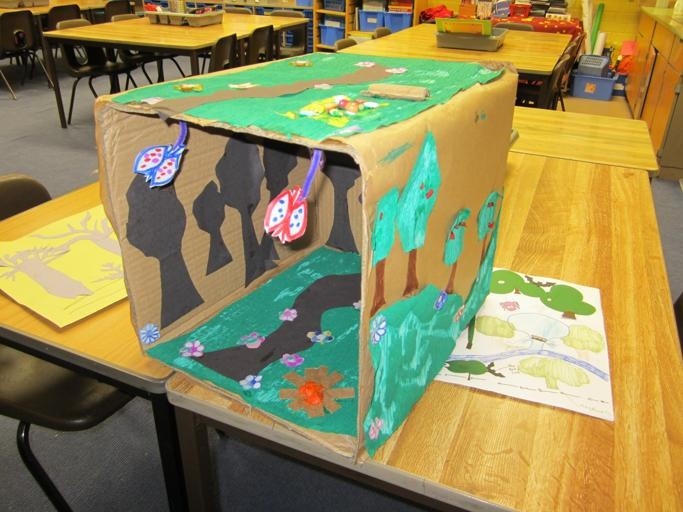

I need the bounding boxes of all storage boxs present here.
[318,23,344,48]
[93,49,519,462]
[571,69,619,101]
[384,12,413,33]
[358,10,385,32]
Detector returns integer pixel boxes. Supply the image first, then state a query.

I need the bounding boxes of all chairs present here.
[0,173,138,512]
[514,32,587,112]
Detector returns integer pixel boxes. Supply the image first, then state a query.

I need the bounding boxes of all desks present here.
[338,22,573,107]
[0,181,186,512]
[508,106,663,177]
[163,150,683,512]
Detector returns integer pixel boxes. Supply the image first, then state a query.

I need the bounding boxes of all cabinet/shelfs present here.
[312,1,355,53]
[626,6,683,184]
